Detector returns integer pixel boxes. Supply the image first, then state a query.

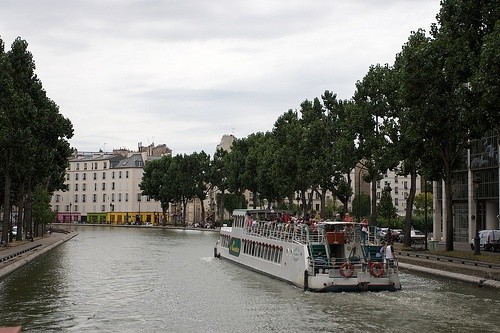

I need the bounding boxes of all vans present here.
[12,226,23,237]
[470,230,500,252]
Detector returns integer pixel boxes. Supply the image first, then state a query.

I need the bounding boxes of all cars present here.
[377,227,425,245]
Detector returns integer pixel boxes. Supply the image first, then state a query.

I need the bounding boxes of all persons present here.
[245,211,334,241]
[359,217,368,244]
[380,240,397,274]
[335,213,344,233]
[344,212,353,243]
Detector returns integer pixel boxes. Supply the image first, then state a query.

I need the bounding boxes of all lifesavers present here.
[370,262,384,277]
[340,262,354,278]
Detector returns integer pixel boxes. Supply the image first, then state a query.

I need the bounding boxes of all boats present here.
[214,209,402,293]
